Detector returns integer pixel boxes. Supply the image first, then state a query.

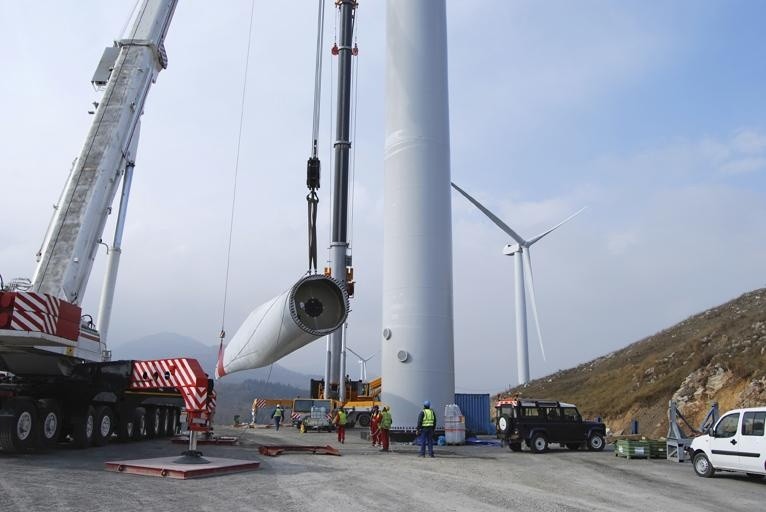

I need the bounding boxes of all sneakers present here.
[418,454,434,458]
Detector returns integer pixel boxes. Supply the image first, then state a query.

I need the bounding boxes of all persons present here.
[335,407,346,444]
[270,404,284,433]
[367,405,393,452]
[415,401,436,458]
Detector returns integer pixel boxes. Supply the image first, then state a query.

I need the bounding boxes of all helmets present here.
[276,404,280,407]
[425,401,430,407]
[384,406,389,411]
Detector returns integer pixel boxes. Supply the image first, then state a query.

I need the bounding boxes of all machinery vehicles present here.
[0,1,216,464]
[252,0,381,434]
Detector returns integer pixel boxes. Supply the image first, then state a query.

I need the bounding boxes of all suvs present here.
[494,397,606,454]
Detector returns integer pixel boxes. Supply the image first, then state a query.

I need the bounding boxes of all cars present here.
[689,407,766,478]
